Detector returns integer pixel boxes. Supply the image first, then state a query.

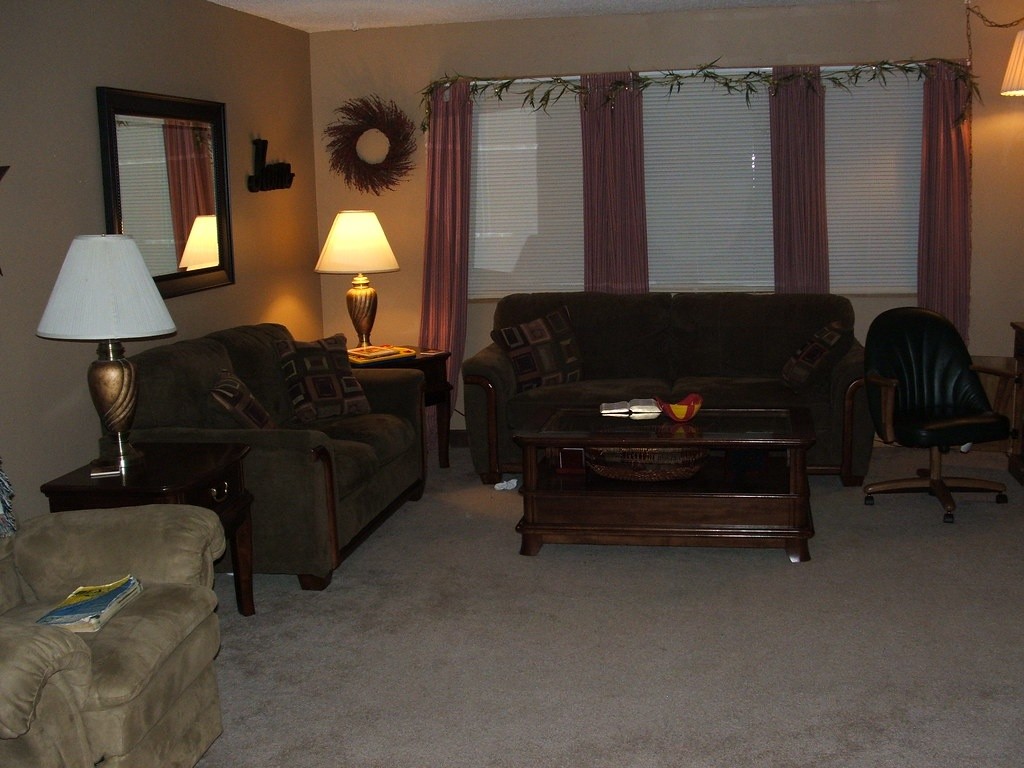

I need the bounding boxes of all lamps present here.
[36,233,177,469]
[179,214,219,271]
[314,210,400,348]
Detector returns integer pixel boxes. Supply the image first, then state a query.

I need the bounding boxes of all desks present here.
[40,443,254,616]
[350,346,453,468]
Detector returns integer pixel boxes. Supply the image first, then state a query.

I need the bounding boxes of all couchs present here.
[460,292,891,488]
[99,323,427,592]
[0,453,227,768]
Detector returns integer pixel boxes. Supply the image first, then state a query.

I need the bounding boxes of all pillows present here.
[207,368,278,430]
[783,319,855,395]
[491,302,589,393]
[273,333,371,424]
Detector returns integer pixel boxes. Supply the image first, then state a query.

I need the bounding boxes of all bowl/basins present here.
[655,404,704,421]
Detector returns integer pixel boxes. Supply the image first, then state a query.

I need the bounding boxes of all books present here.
[35,573,144,631]
[599,399,663,413]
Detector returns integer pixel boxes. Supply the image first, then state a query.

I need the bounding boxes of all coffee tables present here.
[513,402,817,563]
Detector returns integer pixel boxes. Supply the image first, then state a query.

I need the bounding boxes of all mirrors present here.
[95,86,236,300]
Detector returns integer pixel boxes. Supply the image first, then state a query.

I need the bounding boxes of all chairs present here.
[863,307,1023,524]
